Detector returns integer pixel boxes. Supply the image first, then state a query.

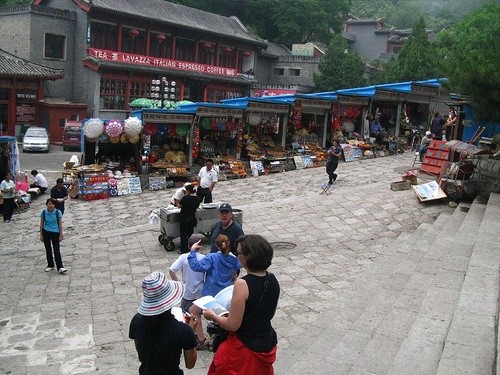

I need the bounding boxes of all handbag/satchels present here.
[207,321,230,351]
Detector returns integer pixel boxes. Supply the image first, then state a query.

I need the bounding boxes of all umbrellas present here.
[81,116,190,144]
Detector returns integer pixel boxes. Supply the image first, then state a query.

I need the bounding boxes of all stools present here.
[321,184,332,195]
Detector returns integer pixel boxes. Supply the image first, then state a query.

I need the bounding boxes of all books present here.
[192,285,235,317]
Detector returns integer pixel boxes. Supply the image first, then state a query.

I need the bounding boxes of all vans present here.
[63,121,82,151]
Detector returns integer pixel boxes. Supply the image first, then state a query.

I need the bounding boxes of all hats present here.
[426,131,432,136]
[137,271,185,316]
[183,182,191,191]
[218,203,232,212]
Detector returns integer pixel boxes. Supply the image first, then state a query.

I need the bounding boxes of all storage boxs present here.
[390,175,417,191]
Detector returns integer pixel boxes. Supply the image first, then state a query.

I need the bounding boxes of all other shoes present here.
[45,267,54,272]
[333,174,337,181]
[58,267,68,274]
[178,251,183,254]
[419,159,422,162]
[195,338,209,350]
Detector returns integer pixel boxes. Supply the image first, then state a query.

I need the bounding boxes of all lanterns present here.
[155,33,167,44]
[224,47,232,55]
[203,42,212,51]
[128,29,140,39]
[242,51,251,57]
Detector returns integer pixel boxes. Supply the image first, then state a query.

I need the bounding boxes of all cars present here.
[21,127,51,153]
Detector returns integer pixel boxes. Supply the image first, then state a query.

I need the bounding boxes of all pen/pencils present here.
[183,309,196,321]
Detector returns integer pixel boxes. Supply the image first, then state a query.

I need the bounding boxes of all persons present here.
[201,235,280,375]
[170,181,198,208]
[187,236,243,348]
[39,198,68,275]
[196,158,218,203]
[177,185,200,254]
[30,169,48,195]
[419,130,435,162]
[128,271,198,375]
[0,172,16,223]
[369,118,384,134]
[445,111,456,127]
[209,203,245,256]
[325,139,342,184]
[51,177,68,238]
[431,112,447,140]
[169,233,206,343]
[450,107,457,116]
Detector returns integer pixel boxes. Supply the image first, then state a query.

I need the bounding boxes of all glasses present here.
[233,251,244,256]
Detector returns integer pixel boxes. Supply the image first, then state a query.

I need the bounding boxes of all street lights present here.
[151,76,175,146]
